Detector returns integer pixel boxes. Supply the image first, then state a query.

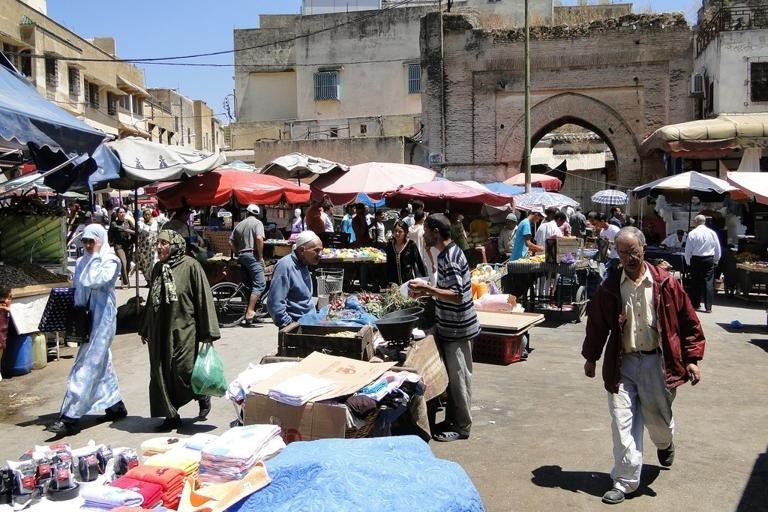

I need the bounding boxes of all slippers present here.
[245,312,264,323]
[429,397,446,407]
[434,430,466,442]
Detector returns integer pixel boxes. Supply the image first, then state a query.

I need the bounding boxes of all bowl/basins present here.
[382,307,424,328]
[375,316,420,342]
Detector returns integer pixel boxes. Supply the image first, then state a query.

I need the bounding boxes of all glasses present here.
[618,250,646,258]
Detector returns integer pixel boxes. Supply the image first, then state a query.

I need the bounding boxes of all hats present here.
[296,230,318,249]
[176,208,194,213]
[530,206,547,218]
[247,204,260,215]
[505,213,517,222]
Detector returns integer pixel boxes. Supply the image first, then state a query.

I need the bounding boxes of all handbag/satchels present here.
[66,306,92,343]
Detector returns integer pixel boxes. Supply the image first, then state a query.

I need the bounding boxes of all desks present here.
[735,261,768,303]
[507,247,599,306]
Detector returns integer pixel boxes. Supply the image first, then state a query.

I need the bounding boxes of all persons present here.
[46,223,126,436]
[580,226,706,504]
[12,187,747,356]
[406,212,482,442]
[137,229,222,432]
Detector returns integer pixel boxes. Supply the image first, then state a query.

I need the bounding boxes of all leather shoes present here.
[657,442,674,466]
[603,489,625,503]
[154,415,180,431]
[200,397,211,417]
[95,409,127,422]
[49,419,82,433]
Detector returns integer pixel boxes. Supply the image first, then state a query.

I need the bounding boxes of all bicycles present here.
[209,258,284,328]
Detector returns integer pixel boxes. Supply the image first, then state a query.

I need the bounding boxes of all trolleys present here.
[504,238,601,324]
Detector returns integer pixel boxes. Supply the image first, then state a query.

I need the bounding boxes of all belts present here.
[238,250,254,254]
[635,348,662,355]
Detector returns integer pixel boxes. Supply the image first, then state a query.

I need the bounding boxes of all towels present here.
[80,423,287,512]
[269,375,337,405]
